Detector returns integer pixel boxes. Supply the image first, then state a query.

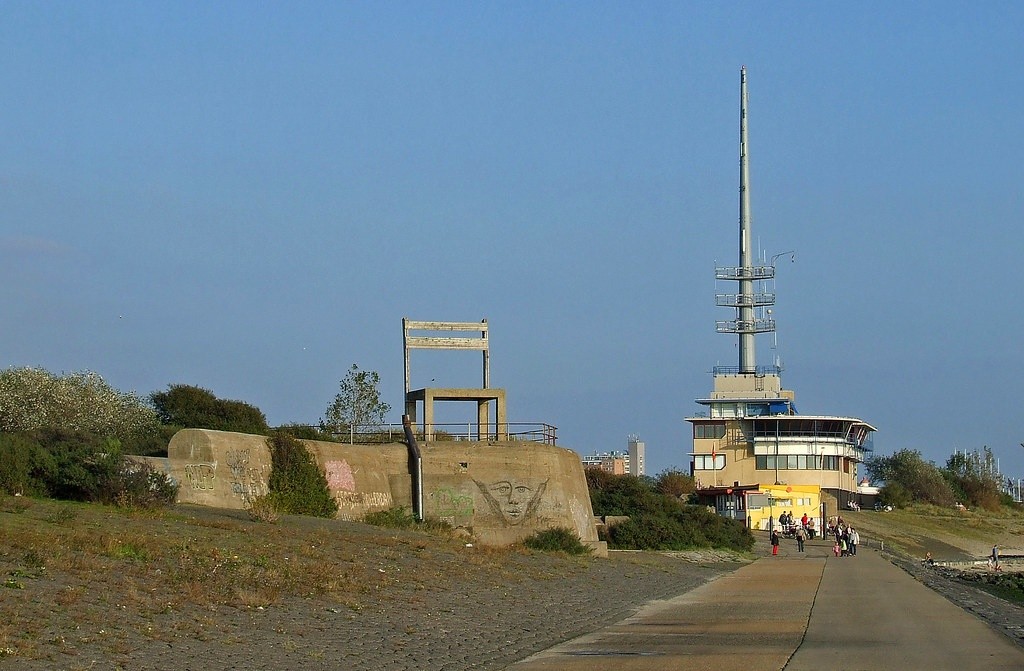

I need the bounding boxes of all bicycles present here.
[781,525,797,539]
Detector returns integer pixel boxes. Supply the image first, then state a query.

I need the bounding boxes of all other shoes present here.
[799,550,800,552]
[772,554,777,555]
[802,550,804,552]
[839,556,842,557]
[854,554,856,556]
[847,553,849,557]
[850,553,852,556]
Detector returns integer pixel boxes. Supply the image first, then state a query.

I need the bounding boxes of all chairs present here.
[401,316,507,441]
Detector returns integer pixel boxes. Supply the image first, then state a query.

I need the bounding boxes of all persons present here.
[780,510,860,557]
[988,545,999,572]
[926,552,933,566]
[848,500,860,512]
[771,529,779,555]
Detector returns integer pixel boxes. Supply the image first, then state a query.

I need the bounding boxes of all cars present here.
[874,501,893,512]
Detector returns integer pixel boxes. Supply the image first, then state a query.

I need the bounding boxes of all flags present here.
[712,445,716,461]
